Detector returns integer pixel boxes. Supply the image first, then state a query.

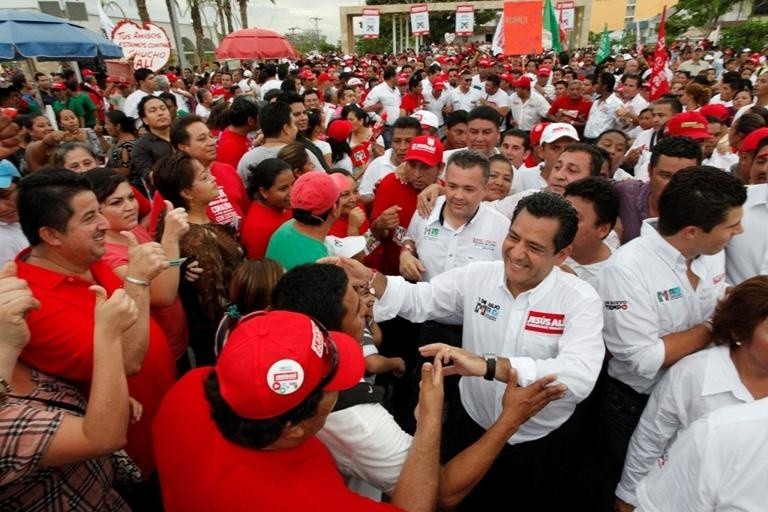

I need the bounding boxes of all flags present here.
[558,4,566,43]
[593,24,611,65]
[542,0,560,54]
[649,5,668,103]
[636,20,644,55]
[490,12,504,57]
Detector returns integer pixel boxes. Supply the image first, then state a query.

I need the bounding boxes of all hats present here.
[215,311,365,420]
[663,104,729,139]
[1,158,21,187]
[732,128,768,154]
[328,120,352,142]
[303,57,551,90]
[82,69,97,76]
[409,110,438,129]
[530,121,580,146]
[404,136,444,167]
[290,173,349,221]
[52,82,65,89]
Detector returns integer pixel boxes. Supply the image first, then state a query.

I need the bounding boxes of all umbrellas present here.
[215,27,298,64]
[0,9,124,88]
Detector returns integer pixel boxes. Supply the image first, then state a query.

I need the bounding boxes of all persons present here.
[1,43,768,512]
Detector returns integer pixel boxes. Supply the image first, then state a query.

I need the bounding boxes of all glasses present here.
[312,317,339,409]
